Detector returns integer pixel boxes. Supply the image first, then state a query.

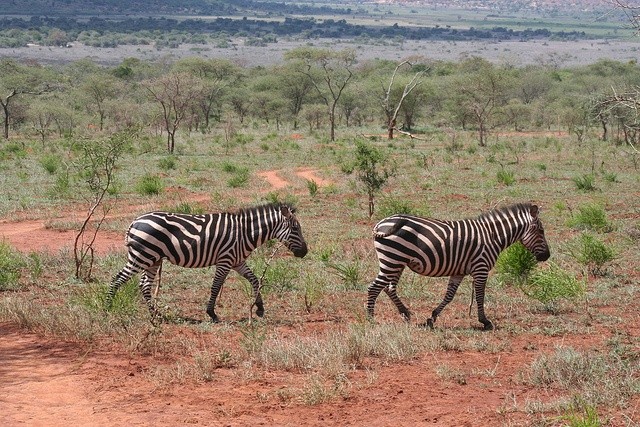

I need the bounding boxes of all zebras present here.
[366,202,550,331]
[102,200,308,329]
